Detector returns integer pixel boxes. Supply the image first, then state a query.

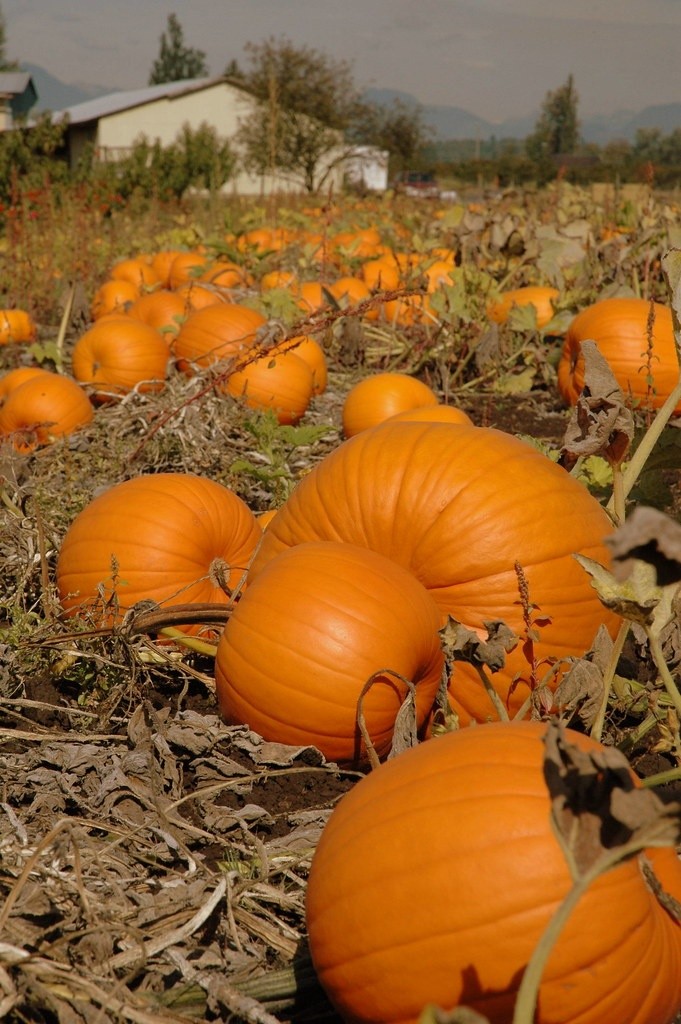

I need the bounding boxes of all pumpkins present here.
[53,425,622,766]
[0,205,681,460]
[303,721,681,1024]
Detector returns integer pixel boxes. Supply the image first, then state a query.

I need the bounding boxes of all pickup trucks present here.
[393,171,440,198]
